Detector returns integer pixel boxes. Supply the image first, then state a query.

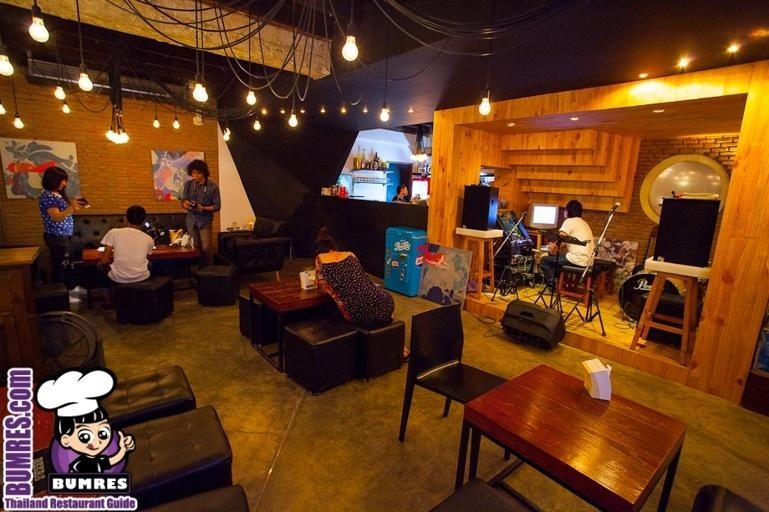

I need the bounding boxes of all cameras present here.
[189,202,198,208]
[78,197,90,208]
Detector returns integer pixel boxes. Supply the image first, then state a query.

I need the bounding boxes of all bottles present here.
[355,149,388,169]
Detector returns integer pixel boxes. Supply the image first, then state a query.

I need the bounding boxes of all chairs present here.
[430,476,529,511]
[399,302,512,482]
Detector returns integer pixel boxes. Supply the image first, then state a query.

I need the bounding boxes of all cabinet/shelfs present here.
[0,246,44,370]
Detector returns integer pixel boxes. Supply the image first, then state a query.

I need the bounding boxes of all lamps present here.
[222,121,231,142]
[61,100,71,114]
[192,1,209,103]
[363,103,369,114]
[1,41,15,77]
[320,103,326,113]
[105,109,129,144]
[54,80,66,100]
[253,116,261,131]
[172,117,180,129]
[153,116,160,128]
[78,2,94,91]
[246,84,257,106]
[379,0,391,122]
[9,0,27,129]
[478,93,492,116]
[29,4,50,43]
[339,98,348,115]
[341,1,359,62]
[0,99,6,115]
[288,108,299,128]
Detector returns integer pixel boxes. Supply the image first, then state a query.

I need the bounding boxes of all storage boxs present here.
[385,226,428,298]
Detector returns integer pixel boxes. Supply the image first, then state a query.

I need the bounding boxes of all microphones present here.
[520,210,528,216]
[607,202,621,212]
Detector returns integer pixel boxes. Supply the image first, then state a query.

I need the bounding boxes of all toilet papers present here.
[582,358,612,401]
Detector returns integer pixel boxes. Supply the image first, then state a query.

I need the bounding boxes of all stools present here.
[629,269,702,365]
[237,286,275,338]
[277,320,360,395]
[198,263,239,306]
[461,237,496,301]
[114,275,175,325]
[97,364,196,429]
[557,265,606,305]
[144,484,250,511]
[33,292,71,354]
[335,314,406,382]
[115,405,233,510]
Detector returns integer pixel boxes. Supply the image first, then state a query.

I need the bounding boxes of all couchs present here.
[71,211,197,283]
[218,216,292,282]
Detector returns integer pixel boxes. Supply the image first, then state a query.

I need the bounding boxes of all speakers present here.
[460,185,500,232]
[652,198,721,268]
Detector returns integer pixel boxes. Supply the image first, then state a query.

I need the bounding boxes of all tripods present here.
[562,210,615,337]
[491,215,525,299]
[534,246,564,313]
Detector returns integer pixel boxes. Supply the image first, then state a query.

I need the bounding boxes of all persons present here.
[392,184,410,202]
[97,205,155,312]
[314,226,410,362]
[540,200,594,295]
[177,159,221,281]
[39,166,89,303]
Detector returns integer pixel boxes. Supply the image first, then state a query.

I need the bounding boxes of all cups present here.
[339,186,346,197]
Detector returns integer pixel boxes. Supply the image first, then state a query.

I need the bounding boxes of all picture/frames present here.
[0,137,80,199]
[417,241,473,313]
[150,149,207,201]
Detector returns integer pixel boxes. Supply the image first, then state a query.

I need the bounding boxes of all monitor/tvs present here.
[525,202,560,231]
